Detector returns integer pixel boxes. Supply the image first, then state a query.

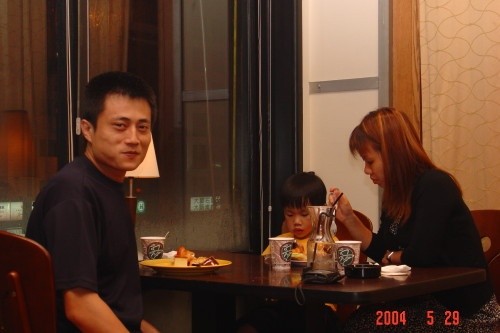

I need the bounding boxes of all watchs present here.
[385,250,395,264]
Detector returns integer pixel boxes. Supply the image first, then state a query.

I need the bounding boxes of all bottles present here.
[307,206,338,285]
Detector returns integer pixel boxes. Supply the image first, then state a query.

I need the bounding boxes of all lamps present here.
[125,132,160,261]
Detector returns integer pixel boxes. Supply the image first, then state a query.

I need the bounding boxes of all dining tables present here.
[139,252,487,333]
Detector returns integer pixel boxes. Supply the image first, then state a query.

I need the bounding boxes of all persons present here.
[260,171,342,264]
[330,106,492,321]
[21,70,163,333]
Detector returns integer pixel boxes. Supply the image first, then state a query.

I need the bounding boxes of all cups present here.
[268,238,294,272]
[346,264,379,281]
[335,240,362,278]
[142,236,165,259]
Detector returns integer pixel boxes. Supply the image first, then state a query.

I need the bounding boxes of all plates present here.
[291,257,308,268]
[139,256,231,276]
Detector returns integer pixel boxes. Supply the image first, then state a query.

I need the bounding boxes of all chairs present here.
[282,209,373,323]
